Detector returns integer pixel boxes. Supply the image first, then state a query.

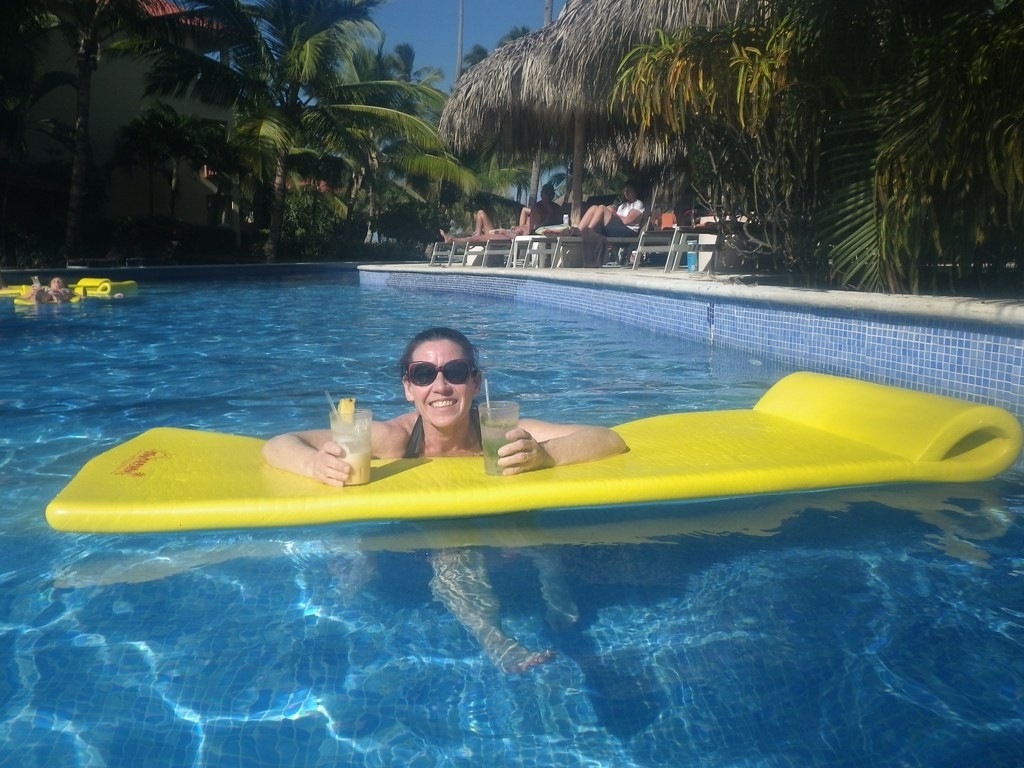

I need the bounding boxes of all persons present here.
[531,185,562,229]
[577,179,645,238]
[20,277,75,303]
[263,327,630,488]
[439,207,534,245]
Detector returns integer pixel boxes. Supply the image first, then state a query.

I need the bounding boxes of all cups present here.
[329,409,372,486]
[477,401,520,475]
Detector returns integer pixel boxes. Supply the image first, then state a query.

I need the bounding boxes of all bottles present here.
[563,214,569,226]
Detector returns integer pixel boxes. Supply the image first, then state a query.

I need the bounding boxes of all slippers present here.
[602,261,621,268]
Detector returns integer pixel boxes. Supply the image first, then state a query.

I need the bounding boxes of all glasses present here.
[408,358,476,388]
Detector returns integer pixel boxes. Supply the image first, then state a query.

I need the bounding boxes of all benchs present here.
[429,241,466,269]
[462,201,586,268]
[632,223,762,275]
[512,194,620,270]
[523,181,660,267]
